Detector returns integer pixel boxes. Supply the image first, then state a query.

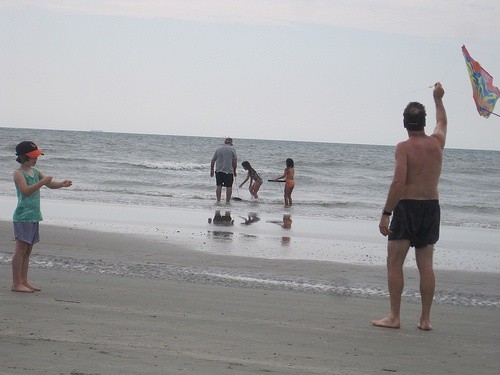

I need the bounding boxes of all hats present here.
[16,141,45,158]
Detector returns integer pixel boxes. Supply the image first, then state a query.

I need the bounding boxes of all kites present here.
[461,43,500,119]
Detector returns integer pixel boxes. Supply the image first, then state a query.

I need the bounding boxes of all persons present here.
[370,83,447,330]
[239,161,263,198]
[273,158,295,205]
[211,138,237,202]
[12,141,72,293]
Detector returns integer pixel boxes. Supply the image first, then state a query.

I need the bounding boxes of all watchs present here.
[381,210,391,216]
